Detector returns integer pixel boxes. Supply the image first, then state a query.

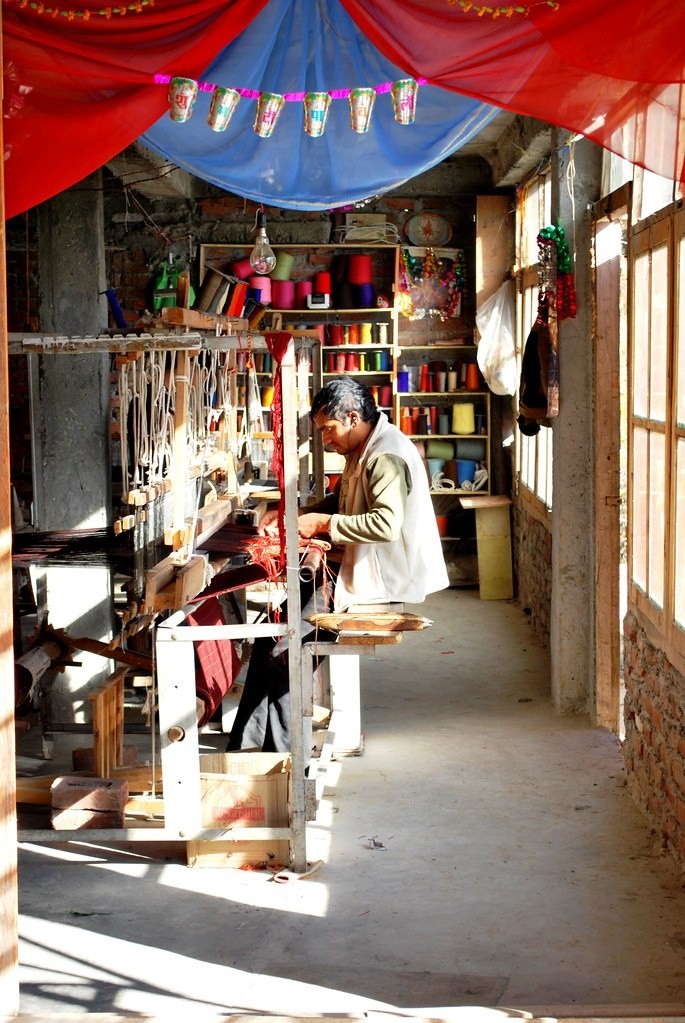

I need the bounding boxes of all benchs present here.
[302,603,406,756]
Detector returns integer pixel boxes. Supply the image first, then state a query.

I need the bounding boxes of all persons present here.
[221,376,450,754]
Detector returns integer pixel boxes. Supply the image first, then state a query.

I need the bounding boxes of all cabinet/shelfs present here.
[459,495,514,600]
[199,243,493,542]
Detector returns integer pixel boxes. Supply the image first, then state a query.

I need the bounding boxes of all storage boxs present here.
[185,752,291,869]
